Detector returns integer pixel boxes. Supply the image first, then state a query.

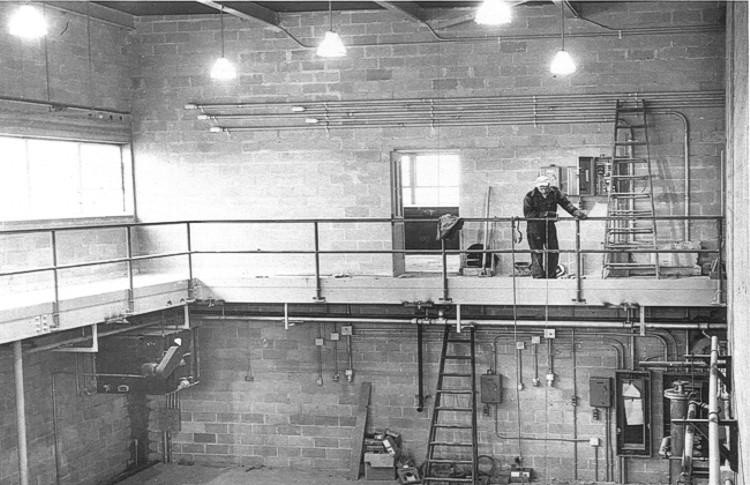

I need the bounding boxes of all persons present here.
[522,175,589,280]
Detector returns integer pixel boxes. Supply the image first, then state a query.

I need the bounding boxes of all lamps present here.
[206,0,239,80]
[315,1,347,59]
[548,2,577,75]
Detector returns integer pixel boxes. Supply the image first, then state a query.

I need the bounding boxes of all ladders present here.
[419,323,479,485]
[601,99,660,280]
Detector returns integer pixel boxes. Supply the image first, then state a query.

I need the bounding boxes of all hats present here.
[534,175,550,187]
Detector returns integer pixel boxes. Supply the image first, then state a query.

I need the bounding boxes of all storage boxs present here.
[365,451,398,480]
